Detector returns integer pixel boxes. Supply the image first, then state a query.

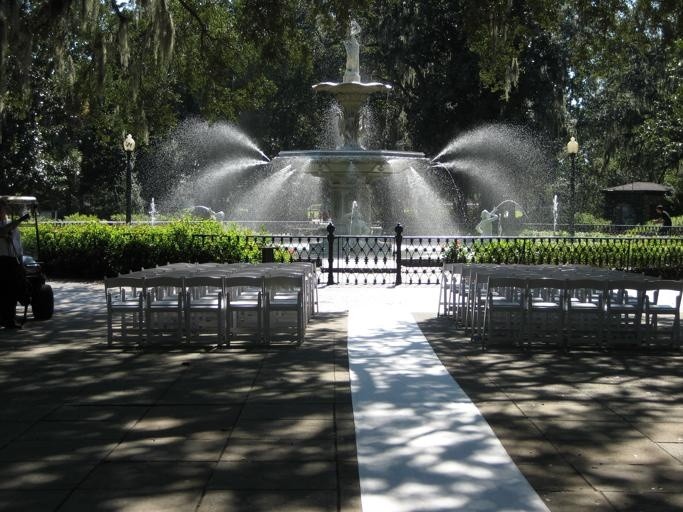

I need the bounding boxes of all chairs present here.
[102,256,318,350]
[436,260,681,351]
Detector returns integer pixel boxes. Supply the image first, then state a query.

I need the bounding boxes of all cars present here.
[173,205,216,221]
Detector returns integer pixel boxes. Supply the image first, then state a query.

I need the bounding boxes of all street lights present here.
[124,134,134,226]
[566,136,579,242]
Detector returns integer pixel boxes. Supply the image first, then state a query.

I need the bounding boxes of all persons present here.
[474,210,498,235]
[650,205,672,236]
[350,206,368,235]
[207,209,225,224]
[0,200,30,329]
